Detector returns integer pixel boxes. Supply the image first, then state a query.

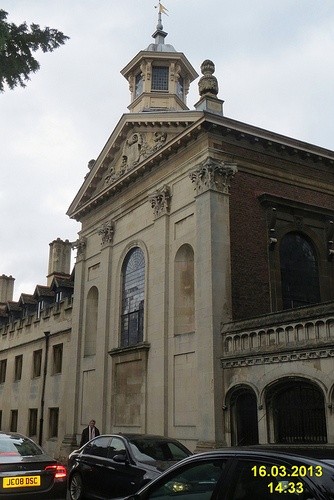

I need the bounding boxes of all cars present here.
[67,432,197,500]
[0,430,66,500]
[118,443,333,500]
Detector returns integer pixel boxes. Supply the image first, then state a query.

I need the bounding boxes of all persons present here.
[79,419,99,448]
